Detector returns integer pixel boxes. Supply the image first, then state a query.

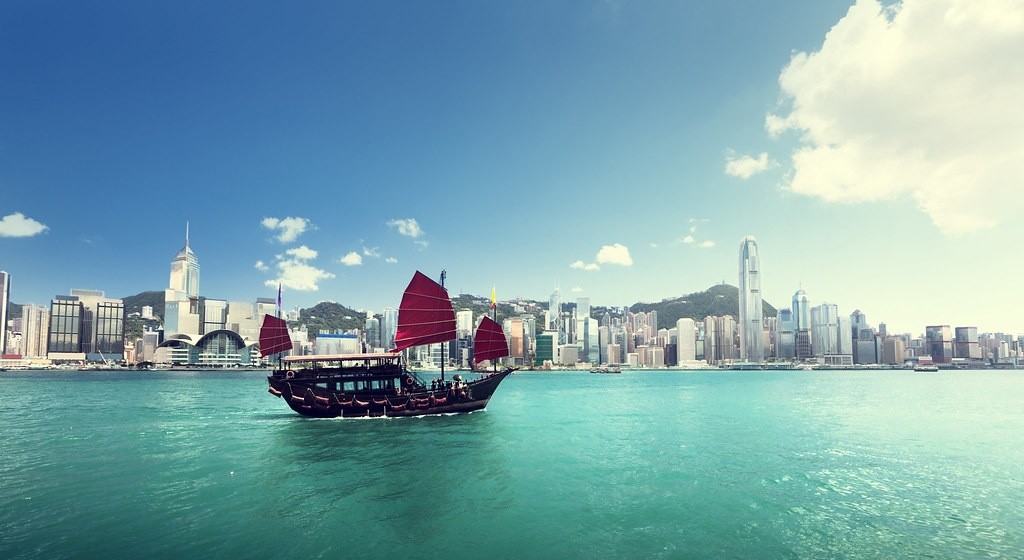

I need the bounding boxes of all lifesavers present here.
[407,378,413,385]
[286,371,295,378]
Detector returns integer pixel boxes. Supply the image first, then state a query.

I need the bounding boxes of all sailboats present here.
[259,269,519,421]
[467,336,500,373]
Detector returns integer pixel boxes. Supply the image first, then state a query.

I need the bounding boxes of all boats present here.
[590,363,621,373]
[913,354,938,372]
